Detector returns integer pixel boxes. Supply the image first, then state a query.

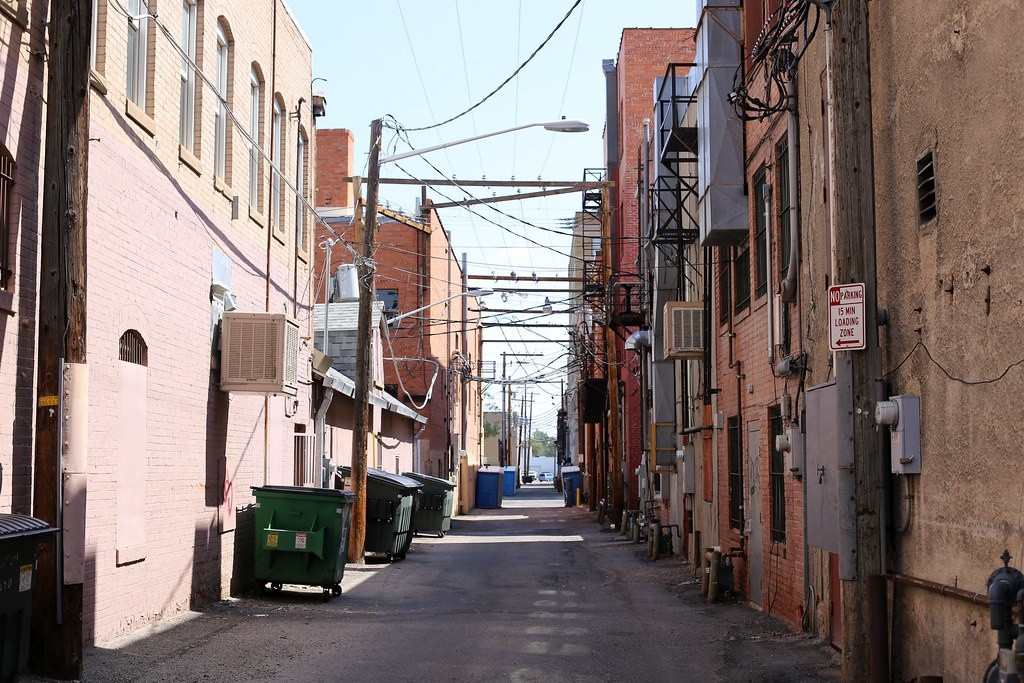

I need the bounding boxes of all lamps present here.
[543,297,552,314]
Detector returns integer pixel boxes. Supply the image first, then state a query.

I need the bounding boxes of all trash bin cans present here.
[561,467,581,504]
[0,512,60,683]
[502,466,517,496]
[401,472,457,538]
[249,485,355,596]
[475,466,504,509]
[336,465,424,561]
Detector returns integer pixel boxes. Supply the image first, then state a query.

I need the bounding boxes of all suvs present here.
[522,471,554,482]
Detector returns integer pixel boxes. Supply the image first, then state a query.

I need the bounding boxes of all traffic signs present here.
[827,282,866,351]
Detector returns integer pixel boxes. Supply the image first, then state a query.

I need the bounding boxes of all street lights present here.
[343,114,590,564]
